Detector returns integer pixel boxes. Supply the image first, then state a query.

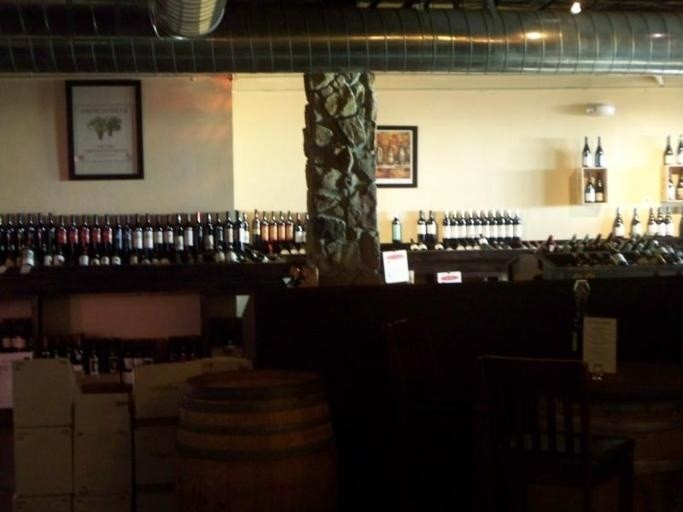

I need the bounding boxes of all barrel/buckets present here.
[173,368,327,512]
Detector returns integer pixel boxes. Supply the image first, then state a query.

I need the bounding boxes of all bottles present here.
[585,172,604,202]
[0,335,187,384]
[581,135,606,167]
[664,135,683,164]
[522,207,683,266]
[0,208,309,269]
[668,169,683,200]
[391,210,401,244]
[408,210,521,251]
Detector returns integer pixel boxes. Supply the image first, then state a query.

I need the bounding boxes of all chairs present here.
[476,354,636,512]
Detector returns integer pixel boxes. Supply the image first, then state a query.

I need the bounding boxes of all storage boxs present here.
[11,358,254,512]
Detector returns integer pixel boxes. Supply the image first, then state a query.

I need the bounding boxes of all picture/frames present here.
[64,79,144,180]
[375,127,416,187]
